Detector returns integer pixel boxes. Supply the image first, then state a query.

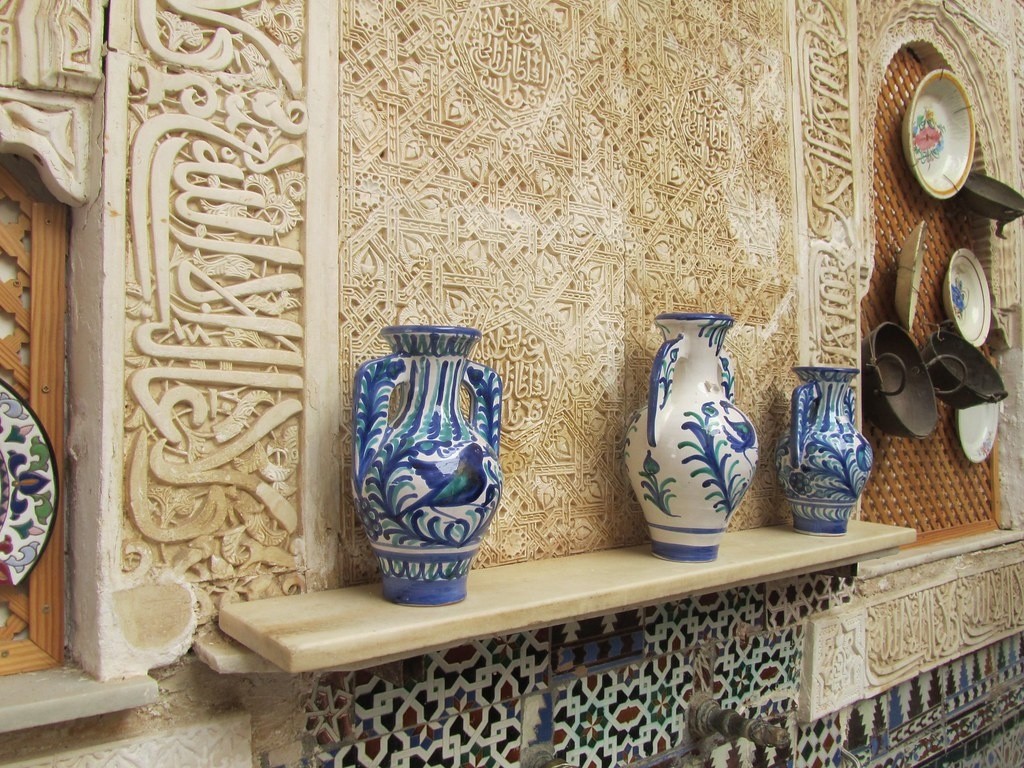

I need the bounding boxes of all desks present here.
[218,519,916,672]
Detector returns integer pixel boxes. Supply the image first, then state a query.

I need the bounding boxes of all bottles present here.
[623,312,758,563]
[772,365,874,535]
[351,325,503,604]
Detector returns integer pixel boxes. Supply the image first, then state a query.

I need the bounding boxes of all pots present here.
[922,319,1008,409]
[861,321,967,440]
[961,173,1024,223]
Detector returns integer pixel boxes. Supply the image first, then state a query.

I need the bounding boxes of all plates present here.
[894,220,928,333]
[942,248,992,347]
[902,68,976,200]
[0,379,59,591]
[954,403,998,463]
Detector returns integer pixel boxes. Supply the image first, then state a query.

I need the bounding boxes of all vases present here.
[623,314,758,564]
[351,324,503,607]
[773,366,873,537]
